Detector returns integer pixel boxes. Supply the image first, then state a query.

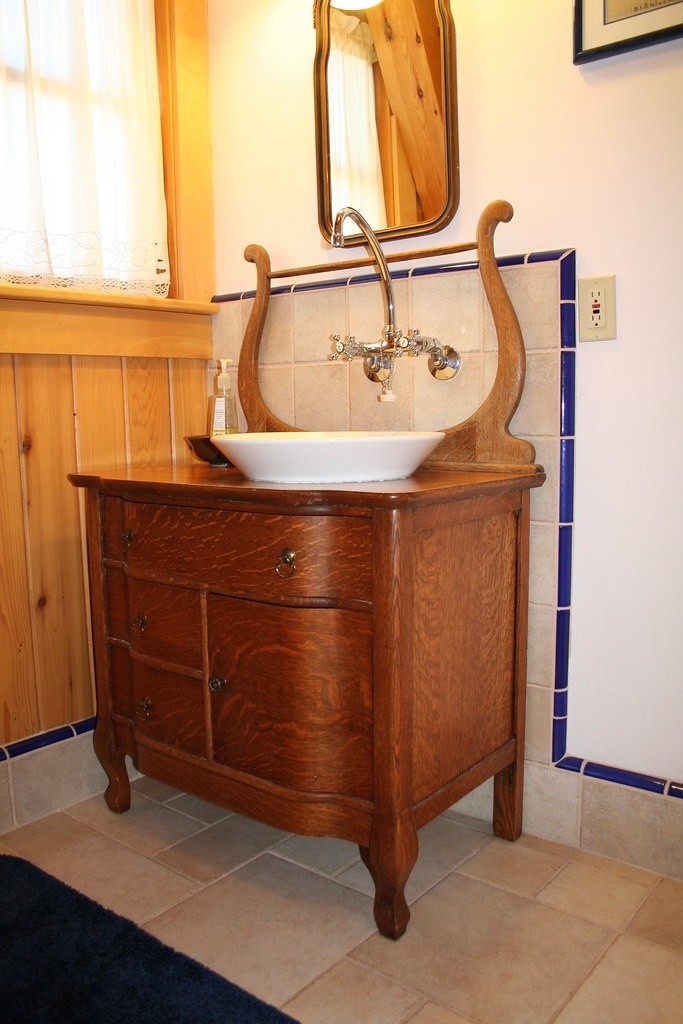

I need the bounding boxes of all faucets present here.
[328,206,460,384]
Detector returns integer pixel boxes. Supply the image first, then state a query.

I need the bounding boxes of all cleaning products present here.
[209,359,240,436]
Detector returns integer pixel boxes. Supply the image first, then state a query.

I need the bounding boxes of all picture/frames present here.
[572,1,682,65]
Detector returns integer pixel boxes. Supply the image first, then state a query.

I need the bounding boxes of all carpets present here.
[1,853,302,1023]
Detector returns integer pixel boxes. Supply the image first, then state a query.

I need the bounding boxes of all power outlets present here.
[577,278,618,344]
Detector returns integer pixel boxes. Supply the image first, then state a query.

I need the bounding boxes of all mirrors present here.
[311,0,459,246]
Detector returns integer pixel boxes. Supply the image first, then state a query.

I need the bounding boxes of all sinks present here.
[210,431,446,485]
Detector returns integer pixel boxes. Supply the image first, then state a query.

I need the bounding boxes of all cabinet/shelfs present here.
[66,459,548,940]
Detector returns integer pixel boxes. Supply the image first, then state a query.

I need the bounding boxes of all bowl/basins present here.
[185,435,231,467]
